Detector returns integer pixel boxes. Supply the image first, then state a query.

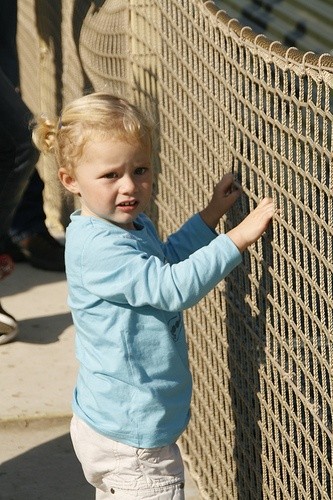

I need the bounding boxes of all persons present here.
[31,93,280,500]
[0,0,65,347]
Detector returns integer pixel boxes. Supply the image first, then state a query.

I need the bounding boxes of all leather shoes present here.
[13,226,67,272]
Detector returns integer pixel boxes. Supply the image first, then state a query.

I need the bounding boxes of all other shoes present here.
[0,303,18,344]
